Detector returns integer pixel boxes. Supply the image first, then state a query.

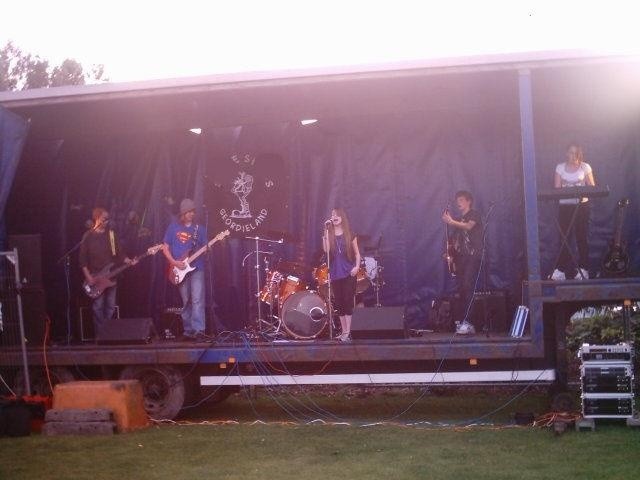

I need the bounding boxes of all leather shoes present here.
[174,333,205,342]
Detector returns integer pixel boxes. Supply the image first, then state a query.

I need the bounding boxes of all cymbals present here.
[268,231,294,241]
[279,259,314,272]
[358,234,370,241]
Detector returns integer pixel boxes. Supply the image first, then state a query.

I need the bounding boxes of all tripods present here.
[239,236,290,341]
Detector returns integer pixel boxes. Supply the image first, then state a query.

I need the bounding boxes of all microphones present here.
[101,214,113,223]
[309,307,324,322]
[326,219,333,225]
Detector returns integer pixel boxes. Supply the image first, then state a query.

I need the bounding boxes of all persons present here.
[163,197,211,340]
[322,206,361,342]
[441,190,481,336]
[79,206,140,341]
[548,142,595,281]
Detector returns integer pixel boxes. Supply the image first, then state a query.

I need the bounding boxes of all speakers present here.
[95,318,161,344]
[0,234,51,346]
[468,287,512,334]
[349,305,410,340]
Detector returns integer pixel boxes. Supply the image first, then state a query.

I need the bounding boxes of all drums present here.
[312,263,330,297]
[355,267,369,294]
[259,270,303,309]
[281,289,329,339]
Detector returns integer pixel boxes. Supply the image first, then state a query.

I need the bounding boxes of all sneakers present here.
[457,323,476,335]
[335,333,352,342]
[549,268,589,280]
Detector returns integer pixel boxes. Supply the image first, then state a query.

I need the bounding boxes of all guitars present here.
[443,201,454,275]
[165,229,230,285]
[602,197,629,273]
[81,244,165,299]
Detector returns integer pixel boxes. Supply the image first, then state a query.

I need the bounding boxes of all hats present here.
[177,198,197,216]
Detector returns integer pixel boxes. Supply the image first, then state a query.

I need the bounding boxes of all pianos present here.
[536,185,612,197]
